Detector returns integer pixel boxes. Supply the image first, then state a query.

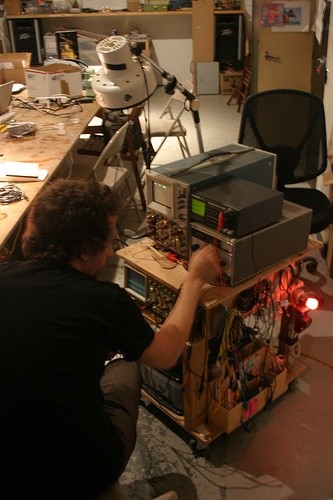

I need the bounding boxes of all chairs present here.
[238,88,333,270]
[0,52,32,116]
[138,96,190,179]
[56,121,144,249]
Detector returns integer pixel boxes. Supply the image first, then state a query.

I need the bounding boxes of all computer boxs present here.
[8,19,43,65]
[214,14,243,62]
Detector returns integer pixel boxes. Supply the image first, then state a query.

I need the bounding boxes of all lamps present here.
[91,36,204,158]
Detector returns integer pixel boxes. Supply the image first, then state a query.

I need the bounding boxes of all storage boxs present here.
[24,65,82,100]
[4,0,22,16]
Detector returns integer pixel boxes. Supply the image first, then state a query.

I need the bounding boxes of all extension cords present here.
[33,97,70,104]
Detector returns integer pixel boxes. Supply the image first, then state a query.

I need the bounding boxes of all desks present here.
[1,97,97,248]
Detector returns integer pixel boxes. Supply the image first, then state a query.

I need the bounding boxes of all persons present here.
[0,181,220,500]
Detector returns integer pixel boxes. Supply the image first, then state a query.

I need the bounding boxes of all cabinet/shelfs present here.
[251,0,313,94]
[4,0,244,95]
